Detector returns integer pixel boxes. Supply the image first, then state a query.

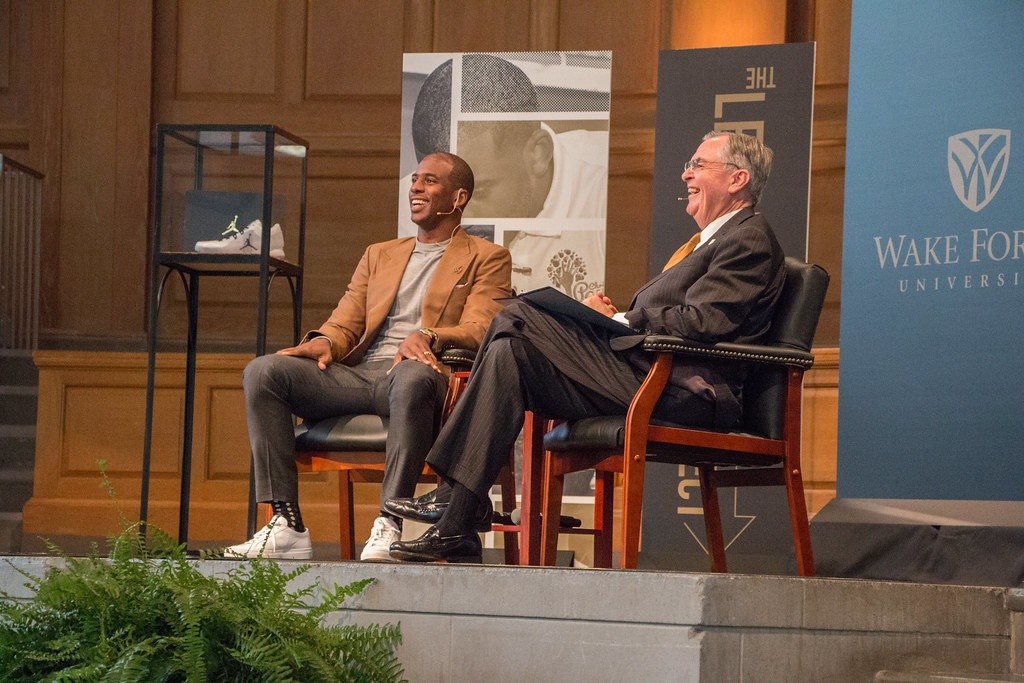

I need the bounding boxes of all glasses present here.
[685,159,739,171]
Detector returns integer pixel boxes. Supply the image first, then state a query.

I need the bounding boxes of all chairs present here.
[267,290,519,565]
[541,256,832,577]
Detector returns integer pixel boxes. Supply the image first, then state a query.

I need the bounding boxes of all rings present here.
[424,351,431,355]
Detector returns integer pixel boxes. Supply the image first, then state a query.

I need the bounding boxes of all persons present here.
[223,152,512,562]
[384,128,786,563]
[398,54,609,301]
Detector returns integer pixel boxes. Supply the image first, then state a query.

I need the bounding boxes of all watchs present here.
[419,328,438,349]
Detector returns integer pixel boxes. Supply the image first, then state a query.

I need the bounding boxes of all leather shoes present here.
[389,525,482,563]
[384,489,493,532]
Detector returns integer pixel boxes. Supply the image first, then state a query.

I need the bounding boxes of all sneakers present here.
[361,517,402,564]
[195,219,285,260]
[224,515,312,559]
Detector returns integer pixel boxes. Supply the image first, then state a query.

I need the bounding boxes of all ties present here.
[662,232,700,273]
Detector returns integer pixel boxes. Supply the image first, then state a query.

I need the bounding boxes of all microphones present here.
[437,188,463,215]
[678,197,689,200]
